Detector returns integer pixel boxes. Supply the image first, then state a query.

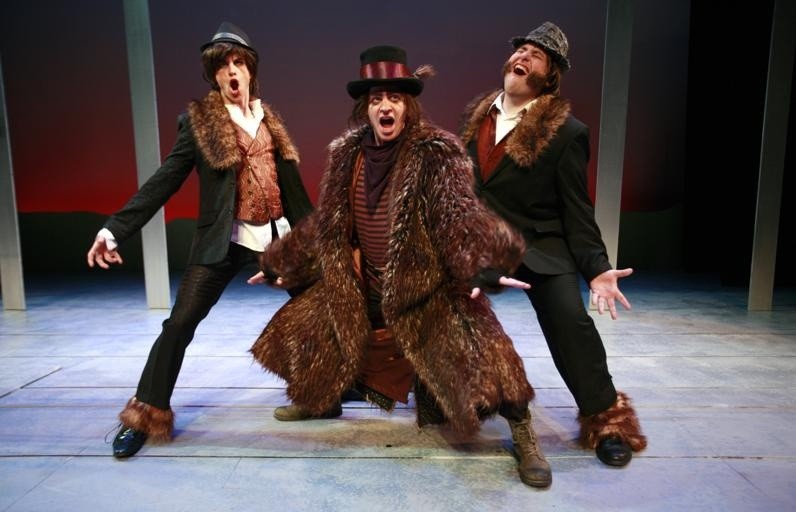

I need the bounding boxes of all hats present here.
[345,44,423,100]
[507,21,572,75]
[199,17,260,62]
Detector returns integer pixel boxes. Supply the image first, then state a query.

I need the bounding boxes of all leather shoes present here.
[110,422,150,459]
[594,432,635,468]
[507,417,553,489]
[272,400,345,423]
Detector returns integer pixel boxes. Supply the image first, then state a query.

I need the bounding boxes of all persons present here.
[455,19,650,467]
[86,21,316,459]
[246,43,554,487]
